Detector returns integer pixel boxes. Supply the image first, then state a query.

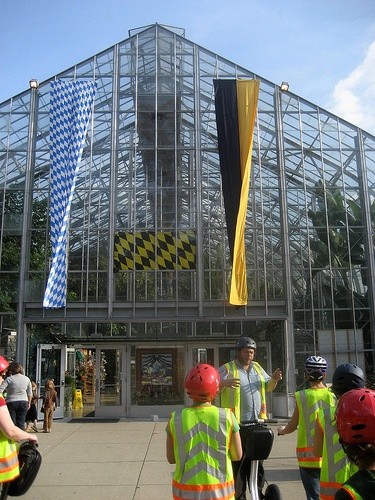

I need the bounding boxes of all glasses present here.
[0,372,6,378]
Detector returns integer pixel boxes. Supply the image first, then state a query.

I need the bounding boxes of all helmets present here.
[185,364,221,402]
[330,388,375,444]
[332,363,365,392]
[305,355,328,379]
[235,336,256,350]
[0,355,11,372]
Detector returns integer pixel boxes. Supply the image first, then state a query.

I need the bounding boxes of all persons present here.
[215,336,282,500]
[277,355,332,500]
[314,363,375,500]
[165,363,243,500]
[0,355,58,500]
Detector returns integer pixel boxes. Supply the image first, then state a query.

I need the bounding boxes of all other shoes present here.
[43,429,52,432]
[31,426,38,432]
[26,428,34,431]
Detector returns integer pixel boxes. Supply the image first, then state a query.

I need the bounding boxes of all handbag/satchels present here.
[5,442,41,496]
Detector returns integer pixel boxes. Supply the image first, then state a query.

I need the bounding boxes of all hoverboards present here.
[1,440,42,500]
[239,419,280,500]
